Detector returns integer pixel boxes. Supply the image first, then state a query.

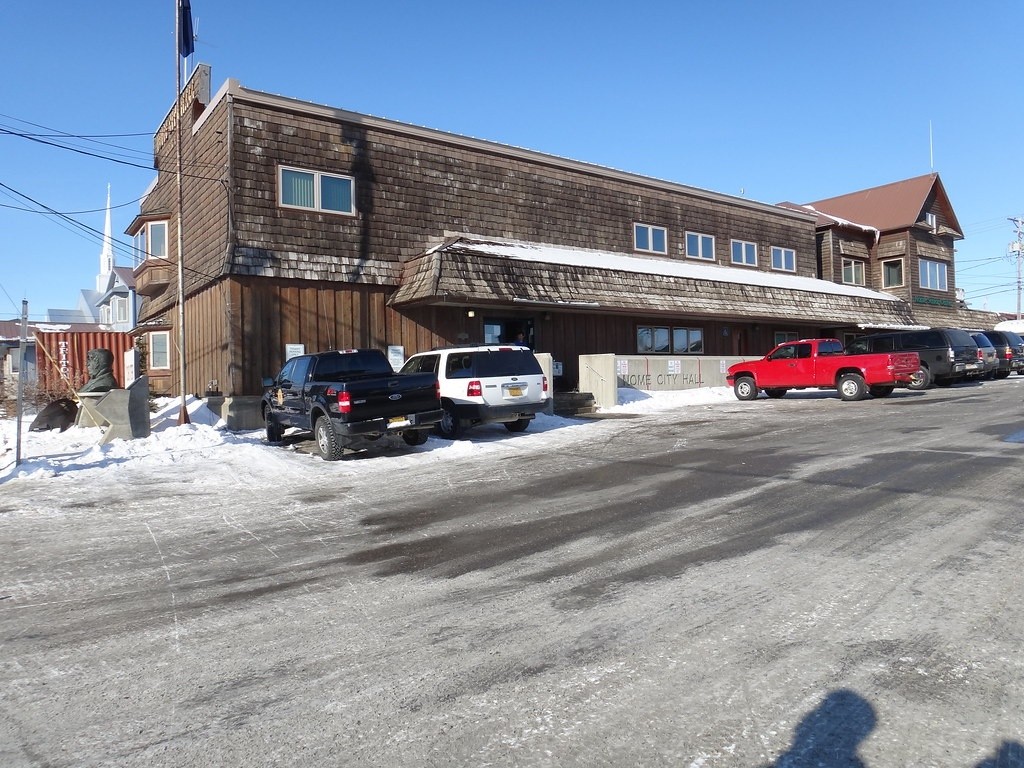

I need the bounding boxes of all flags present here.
[177,0,196,59]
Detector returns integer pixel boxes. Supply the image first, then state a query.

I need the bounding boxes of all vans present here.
[985,331,1024,380]
[968,333,999,385]
[844,327,978,390]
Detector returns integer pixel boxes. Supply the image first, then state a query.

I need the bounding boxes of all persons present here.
[78,348,119,392]
[514,332,528,346]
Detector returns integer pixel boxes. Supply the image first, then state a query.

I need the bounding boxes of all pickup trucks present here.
[726,338,921,401]
[260,348,444,461]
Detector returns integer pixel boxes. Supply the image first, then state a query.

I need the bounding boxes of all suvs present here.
[396,345,549,439]
